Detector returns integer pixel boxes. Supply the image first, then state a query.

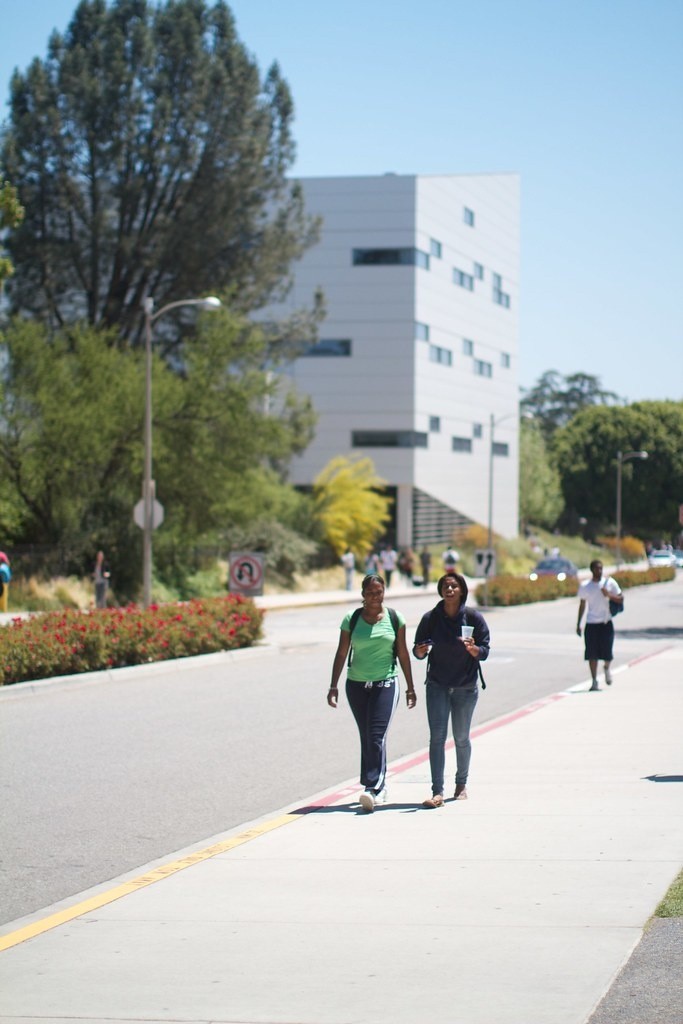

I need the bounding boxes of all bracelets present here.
[405,690,415,694]
[328,687,338,690]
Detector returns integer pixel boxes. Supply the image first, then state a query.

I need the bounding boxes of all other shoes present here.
[589,681,599,690]
[423,795,445,808]
[359,791,375,811]
[374,789,386,803]
[454,783,467,799]
[605,665,612,684]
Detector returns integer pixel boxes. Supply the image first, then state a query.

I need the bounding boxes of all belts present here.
[351,678,394,688]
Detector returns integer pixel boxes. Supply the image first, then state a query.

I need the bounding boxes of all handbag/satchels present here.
[603,577,624,616]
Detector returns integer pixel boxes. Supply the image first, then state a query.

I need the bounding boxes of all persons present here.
[576,559,623,692]
[367,544,431,589]
[342,547,354,590]
[412,572,490,809]
[326,574,417,813]
[90,551,112,608]
[648,539,681,552]
[442,546,458,573]
[0,551,11,614]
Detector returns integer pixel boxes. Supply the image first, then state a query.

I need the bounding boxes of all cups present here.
[461,625,474,643]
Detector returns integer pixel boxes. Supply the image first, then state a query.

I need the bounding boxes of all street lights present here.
[141,296,224,605]
[615,451,649,570]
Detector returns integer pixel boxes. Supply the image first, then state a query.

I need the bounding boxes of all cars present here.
[531,555,579,581]
[649,548,683,571]
